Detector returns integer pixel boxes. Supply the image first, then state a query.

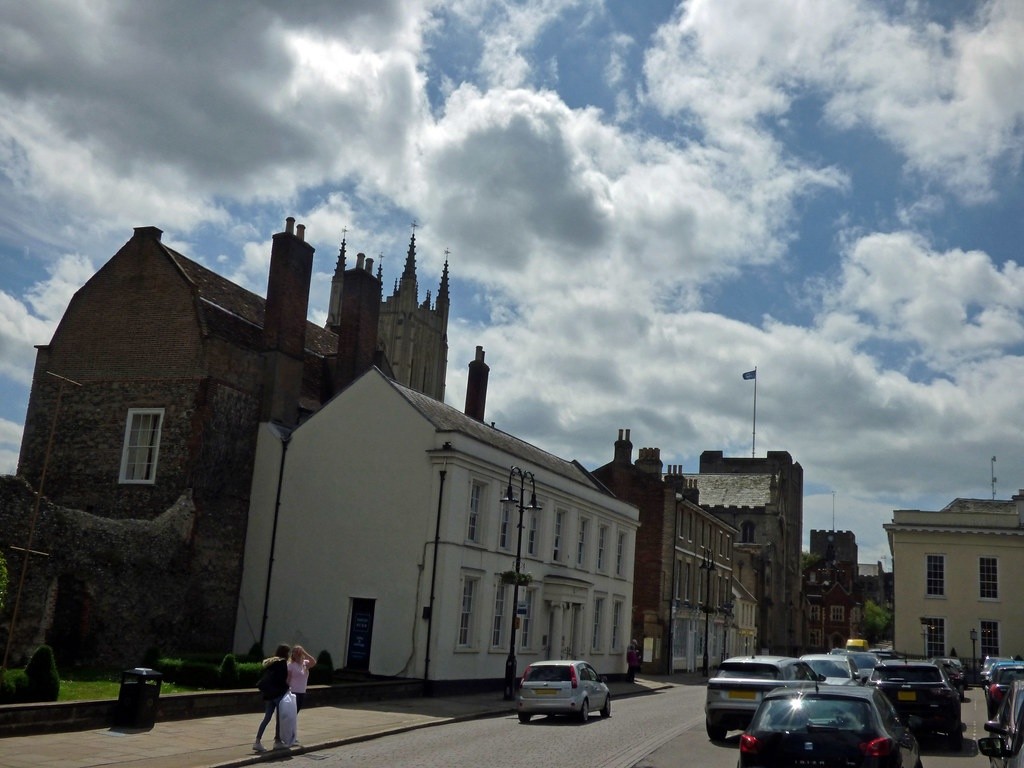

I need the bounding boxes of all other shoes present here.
[293,740,300,745]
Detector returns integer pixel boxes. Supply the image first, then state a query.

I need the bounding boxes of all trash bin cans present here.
[114,668,163,729]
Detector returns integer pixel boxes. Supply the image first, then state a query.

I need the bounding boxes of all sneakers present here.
[273,742,289,749]
[253,743,267,751]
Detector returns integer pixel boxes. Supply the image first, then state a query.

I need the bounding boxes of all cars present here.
[829,646,1024,715]
[704,655,829,742]
[867,658,963,751]
[978,679,1024,768]
[791,653,865,686]
[514,660,612,724]
[737,684,924,768]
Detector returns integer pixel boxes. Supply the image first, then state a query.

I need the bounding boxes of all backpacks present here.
[255,668,275,693]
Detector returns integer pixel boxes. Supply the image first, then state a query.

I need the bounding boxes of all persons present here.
[635,650,642,671]
[625,639,637,683]
[286,646,316,714]
[252,644,290,750]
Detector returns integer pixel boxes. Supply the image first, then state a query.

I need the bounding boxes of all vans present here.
[846,638,870,652]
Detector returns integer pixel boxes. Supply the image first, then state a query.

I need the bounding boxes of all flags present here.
[743,370,756,380]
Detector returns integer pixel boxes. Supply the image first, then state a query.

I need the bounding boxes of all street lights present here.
[500,465,543,702]
[700,547,718,678]
